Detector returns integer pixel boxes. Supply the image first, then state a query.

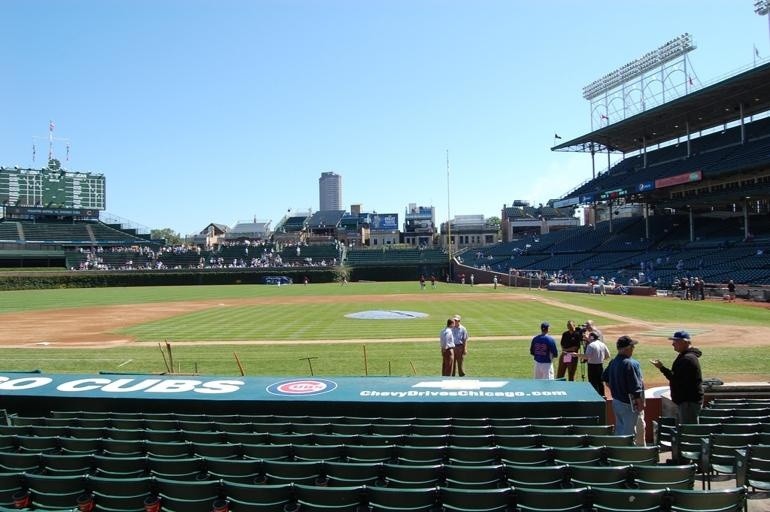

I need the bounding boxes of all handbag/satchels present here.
[702,378,723,387]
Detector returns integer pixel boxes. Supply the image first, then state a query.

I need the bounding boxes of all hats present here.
[617,336,638,348]
[541,323,549,330]
[453,315,461,321]
[669,331,691,341]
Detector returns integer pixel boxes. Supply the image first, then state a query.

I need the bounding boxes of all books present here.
[629,390,646,413]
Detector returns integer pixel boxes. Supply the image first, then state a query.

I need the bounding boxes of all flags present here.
[49,120,56,132]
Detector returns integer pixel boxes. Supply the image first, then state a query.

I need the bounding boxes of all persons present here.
[67,221,770,288]
[601,334,648,447]
[580,319,605,364]
[648,330,706,464]
[569,331,611,396]
[439,318,456,376]
[529,321,560,379]
[451,314,469,377]
[556,320,580,381]
